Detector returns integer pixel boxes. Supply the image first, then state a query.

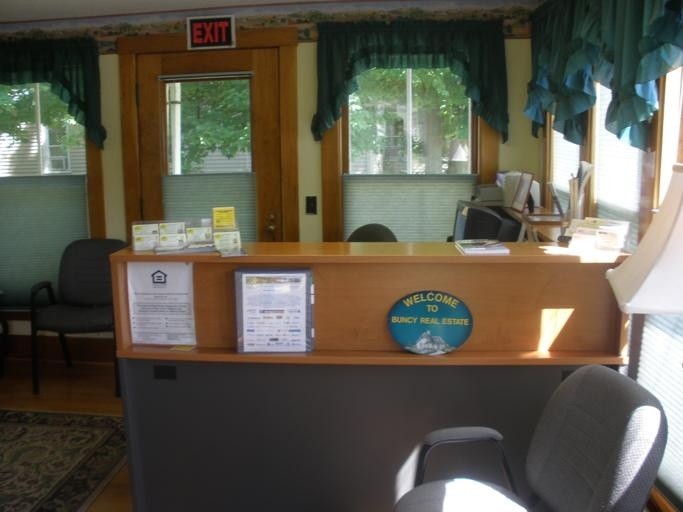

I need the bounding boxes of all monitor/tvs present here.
[451,200,504,240]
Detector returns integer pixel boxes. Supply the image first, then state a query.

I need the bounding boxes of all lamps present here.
[606,164,683,317]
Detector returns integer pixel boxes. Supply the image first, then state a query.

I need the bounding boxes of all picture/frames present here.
[233,268,314,353]
[511,170,534,212]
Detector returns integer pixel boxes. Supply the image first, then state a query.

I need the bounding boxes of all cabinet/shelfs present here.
[111,196,636,511]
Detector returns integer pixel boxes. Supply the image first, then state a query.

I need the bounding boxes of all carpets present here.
[1,408,130,511]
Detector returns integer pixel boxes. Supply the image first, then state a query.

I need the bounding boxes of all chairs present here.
[345,223,399,242]
[392,363,668,511]
[30,238,129,396]
